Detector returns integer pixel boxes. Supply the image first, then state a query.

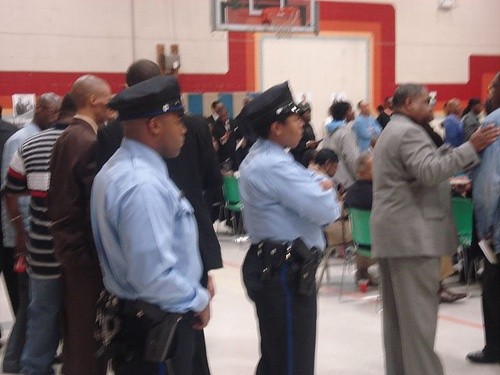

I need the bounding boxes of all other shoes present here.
[466,345,500,364]
[356,272,378,286]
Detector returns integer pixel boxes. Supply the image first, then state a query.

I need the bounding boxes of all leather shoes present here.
[439,286,467,305]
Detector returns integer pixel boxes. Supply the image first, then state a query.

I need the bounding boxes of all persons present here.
[371,84,499,375]
[235,80,342,375]
[0,58,499,375]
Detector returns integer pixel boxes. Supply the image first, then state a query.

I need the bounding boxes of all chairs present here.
[316,219,353,291]
[338,208,383,309]
[451,197,484,298]
[219,176,246,241]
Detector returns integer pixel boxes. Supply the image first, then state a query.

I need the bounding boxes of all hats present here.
[103,74,186,122]
[236,81,310,128]
